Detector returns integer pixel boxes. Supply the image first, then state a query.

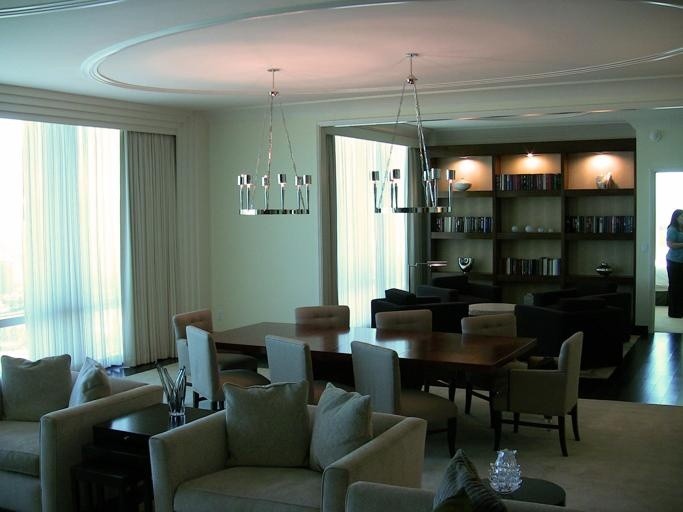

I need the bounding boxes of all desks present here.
[480,476,566,507]
[466,303,517,316]
[209,321,537,370]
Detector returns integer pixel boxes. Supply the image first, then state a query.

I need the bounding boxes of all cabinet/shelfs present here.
[408,137,636,325]
[93,402,221,512]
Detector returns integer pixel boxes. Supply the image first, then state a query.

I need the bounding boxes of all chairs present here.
[460,316,516,415]
[490,331,584,457]
[185,325,271,411]
[172,309,257,387]
[295,306,350,328]
[375,309,456,403]
[265,334,355,406]
[351,341,458,458]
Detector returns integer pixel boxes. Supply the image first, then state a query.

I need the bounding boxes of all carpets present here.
[117,360,683,512]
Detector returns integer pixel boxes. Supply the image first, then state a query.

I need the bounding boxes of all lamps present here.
[372,53,456,213]
[408,260,449,294]
[237,68,311,215]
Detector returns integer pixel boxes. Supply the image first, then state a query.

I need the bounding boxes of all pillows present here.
[311,382,372,473]
[1,355,73,421]
[222,378,311,468]
[432,448,508,511]
[69,356,110,408]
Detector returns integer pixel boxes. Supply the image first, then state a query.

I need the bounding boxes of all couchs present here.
[0,370,164,512]
[513,298,624,372]
[149,405,427,512]
[532,289,633,344]
[344,481,581,512]
[432,274,506,304]
[371,288,469,335]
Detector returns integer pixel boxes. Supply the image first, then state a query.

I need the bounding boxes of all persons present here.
[665,209,683,318]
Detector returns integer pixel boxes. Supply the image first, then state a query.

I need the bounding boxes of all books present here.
[495,173,561,191]
[565,215,634,234]
[497,256,561,276]
[431,217,494,234]
